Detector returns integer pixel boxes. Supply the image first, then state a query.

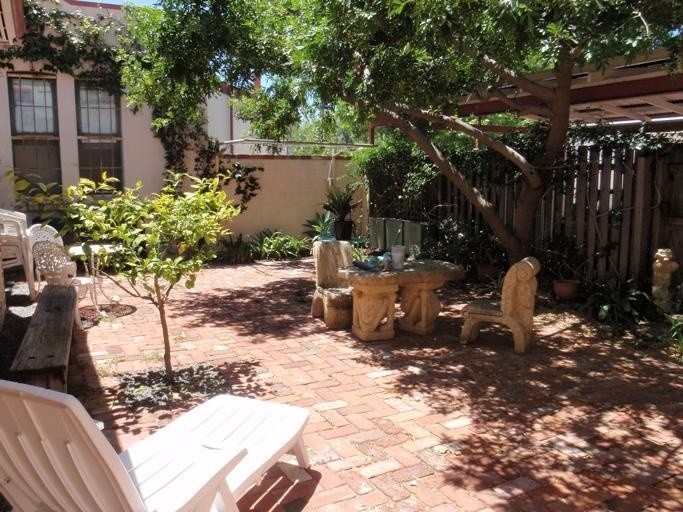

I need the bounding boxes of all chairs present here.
[0,209,106,330]
[460,257,540,354]
[0,380,311,512]
[309,239,351,330]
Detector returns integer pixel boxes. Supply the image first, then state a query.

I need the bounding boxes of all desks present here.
[68,244,124,318]
[335,259,464,341]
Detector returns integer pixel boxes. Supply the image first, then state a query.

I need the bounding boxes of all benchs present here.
[10,285,78,393]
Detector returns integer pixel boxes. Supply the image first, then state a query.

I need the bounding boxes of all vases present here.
[552,280,581,300]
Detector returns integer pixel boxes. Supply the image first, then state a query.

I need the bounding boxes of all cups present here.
[390,245,405,269]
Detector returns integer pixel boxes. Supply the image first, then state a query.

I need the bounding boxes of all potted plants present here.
[321,185,363,239]
